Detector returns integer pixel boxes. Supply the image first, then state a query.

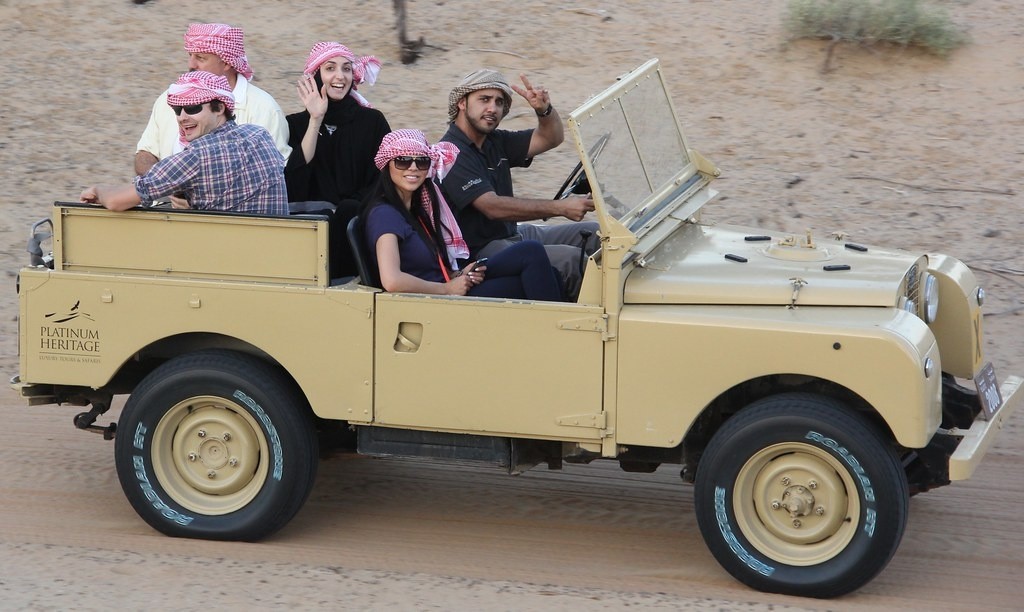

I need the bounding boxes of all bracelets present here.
[309,119,322,134]
[534,105,553,116]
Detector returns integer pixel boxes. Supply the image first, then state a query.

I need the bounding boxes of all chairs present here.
[347,216,386,291]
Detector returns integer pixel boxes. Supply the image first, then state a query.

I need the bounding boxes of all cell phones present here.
[466,258,488,276]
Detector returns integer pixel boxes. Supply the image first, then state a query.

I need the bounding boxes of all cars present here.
[8,58,1024,601]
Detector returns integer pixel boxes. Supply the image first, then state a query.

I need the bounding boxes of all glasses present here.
[171,101,211,116]
[391,156,431,170]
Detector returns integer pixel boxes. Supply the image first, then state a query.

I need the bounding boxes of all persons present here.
[135,24,294,180]
[285,43,392,278]
[433,71,601,302]
[366,129,564,302]
[80,72,289,216]
